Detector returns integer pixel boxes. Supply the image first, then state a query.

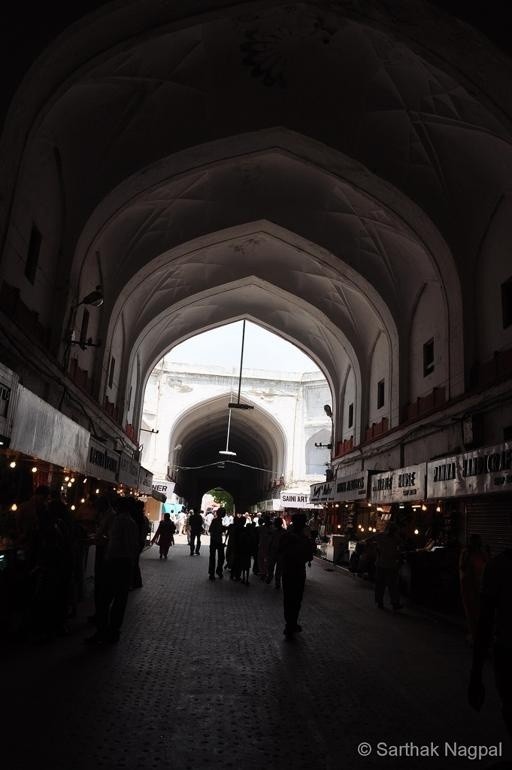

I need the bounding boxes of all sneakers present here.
[80,614,119,647]
[158,551,281,587]
[281,620,305,645]
[374,600,405,612]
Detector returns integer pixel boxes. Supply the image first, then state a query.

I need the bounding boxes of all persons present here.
[171,508,323,588]
[1,450,150,649]
[350,521,493,644]
[151,513,176,561]
[276,510,315,640]
[467,547,511,770]
